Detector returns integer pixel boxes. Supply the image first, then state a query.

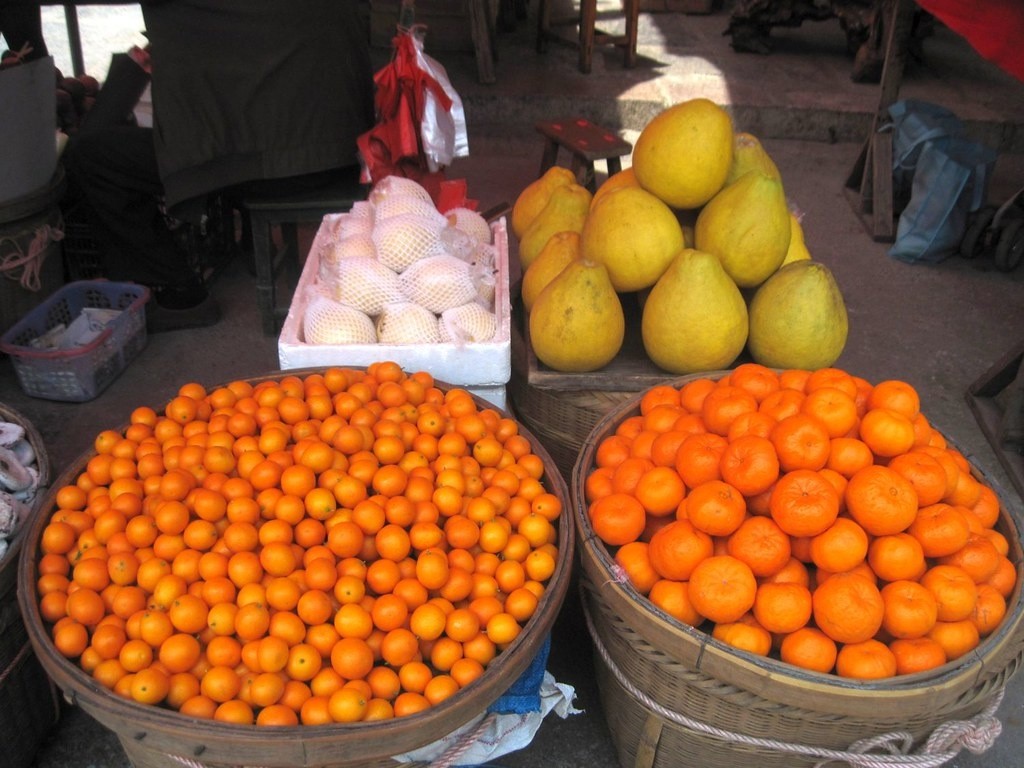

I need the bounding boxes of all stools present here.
[536,117,631,197]
[244,175,371,335]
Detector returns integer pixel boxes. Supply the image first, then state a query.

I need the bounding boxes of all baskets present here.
[3,279,153,400]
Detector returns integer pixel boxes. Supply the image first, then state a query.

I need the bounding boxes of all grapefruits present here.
[510,98,849,373]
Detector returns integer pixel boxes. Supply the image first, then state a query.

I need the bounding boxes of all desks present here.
[843,1,1024,244]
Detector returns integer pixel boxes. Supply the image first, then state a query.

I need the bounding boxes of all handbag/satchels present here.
[885,136,996,263]
[879,97,962,210]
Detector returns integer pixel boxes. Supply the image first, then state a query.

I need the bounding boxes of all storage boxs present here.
[278,213,511,413]
[0,279,152,402]
[62,188,239,299]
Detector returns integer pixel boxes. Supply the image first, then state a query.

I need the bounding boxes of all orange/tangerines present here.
[585,362,1016,682]
[38,358,564,728]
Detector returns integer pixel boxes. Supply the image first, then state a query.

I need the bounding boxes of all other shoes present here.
[144,291,221,334]
